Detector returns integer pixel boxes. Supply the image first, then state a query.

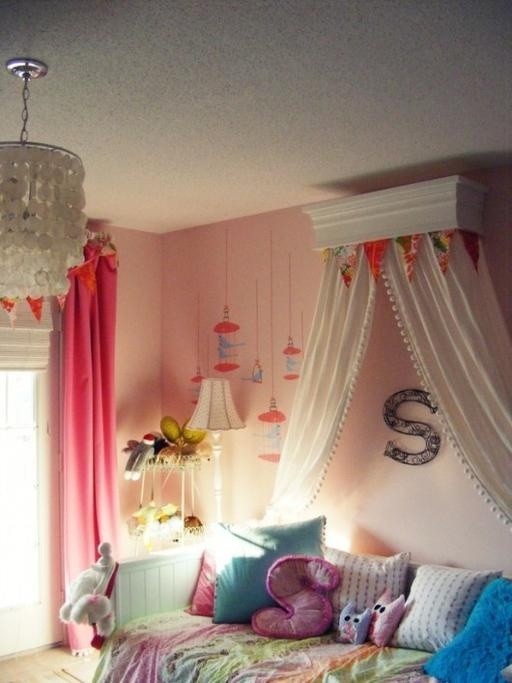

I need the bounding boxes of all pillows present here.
[326,548,411,631]
[186,516,326,623]
[389,565,504,652]
[424,578,511,683]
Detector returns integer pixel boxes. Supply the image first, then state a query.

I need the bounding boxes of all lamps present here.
[0,56,88,300]
[184,375,247,532]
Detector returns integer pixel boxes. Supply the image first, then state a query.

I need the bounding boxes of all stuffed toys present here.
[59,542,116,638]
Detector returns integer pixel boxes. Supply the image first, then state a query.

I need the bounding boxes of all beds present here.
[102,541,512,683]
